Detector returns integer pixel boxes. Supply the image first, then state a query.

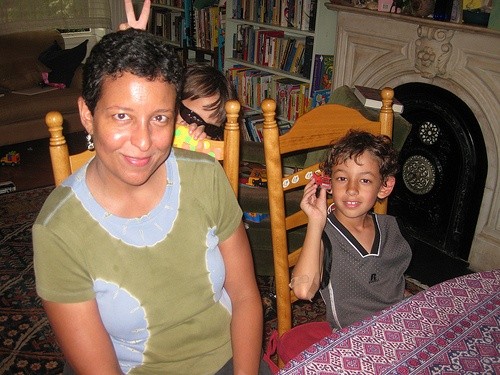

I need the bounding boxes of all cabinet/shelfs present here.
[232,0,339,125]
[132,0,226,73]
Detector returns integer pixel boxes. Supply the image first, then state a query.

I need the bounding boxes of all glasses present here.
[179,101,223,139]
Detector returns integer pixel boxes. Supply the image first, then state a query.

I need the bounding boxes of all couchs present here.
[0,24,108,148]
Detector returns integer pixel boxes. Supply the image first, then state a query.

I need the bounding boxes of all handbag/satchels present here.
[273,320,334,364]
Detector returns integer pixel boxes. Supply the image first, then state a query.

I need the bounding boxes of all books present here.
[231,0,317,32]
[233,24,314,79]
[132,0,226,73]
[226,65,310,142]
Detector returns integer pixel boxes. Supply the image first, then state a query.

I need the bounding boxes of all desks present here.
[279,269,500,375]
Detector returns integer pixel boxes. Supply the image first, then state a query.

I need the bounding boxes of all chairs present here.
[265,85,394,371]
[44,100,241,191]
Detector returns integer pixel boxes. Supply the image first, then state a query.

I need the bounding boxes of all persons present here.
[31,27,271,375]
[291,129,412,331]
[121,0,234,141]
